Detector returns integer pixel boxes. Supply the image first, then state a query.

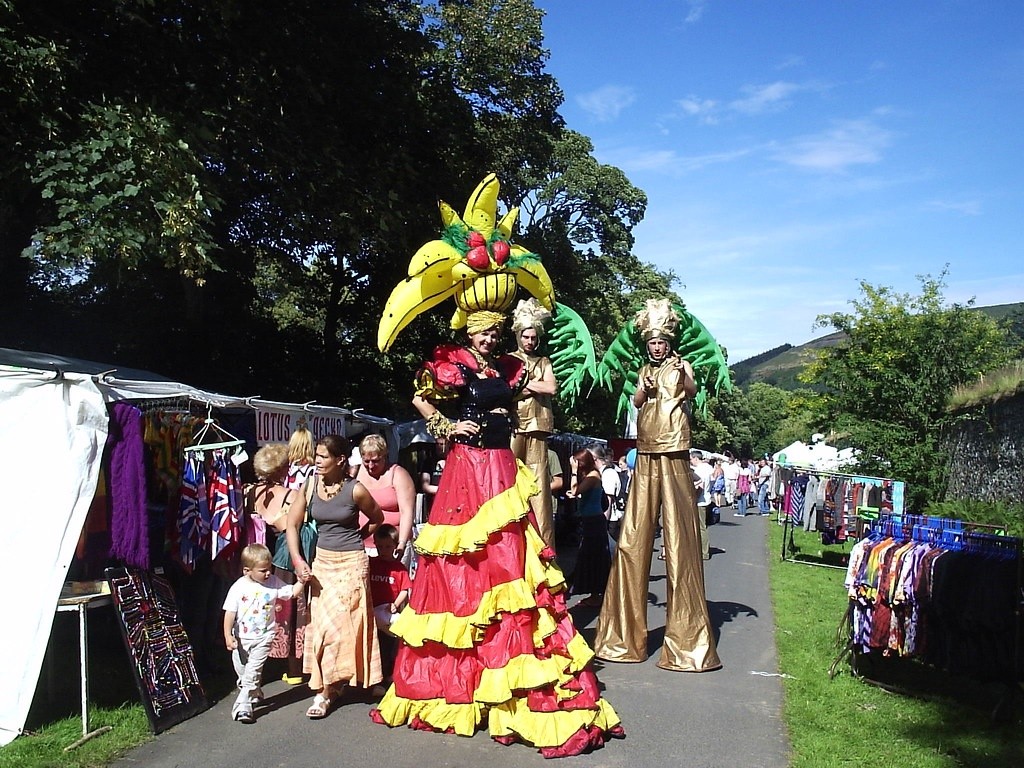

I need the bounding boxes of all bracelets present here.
[425,407,458,440]
[398,540,406,546]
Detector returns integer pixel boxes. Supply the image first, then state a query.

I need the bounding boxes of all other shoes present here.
[281,673,303,684]
[237,711,253,721]
[734,513,745,517]
[372,685,387,698]
[657,553,666,560]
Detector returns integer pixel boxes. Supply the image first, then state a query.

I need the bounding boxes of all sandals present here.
[306,691,338,716]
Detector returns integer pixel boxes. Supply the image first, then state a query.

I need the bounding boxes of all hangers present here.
[869,509,1024,564]
[137,397,247,462]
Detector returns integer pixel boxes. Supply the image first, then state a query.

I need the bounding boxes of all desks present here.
[43,594,113,753]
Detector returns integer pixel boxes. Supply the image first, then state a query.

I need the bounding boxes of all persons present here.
[501,318,560,571]
[553,443,637,609]
[658,450,772,560]
[593,296,723,673]
[148,426,451,722]
[411,311,534,728]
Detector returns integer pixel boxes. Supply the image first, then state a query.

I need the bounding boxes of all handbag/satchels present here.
[272,474,319,572]
[601,486,610,513]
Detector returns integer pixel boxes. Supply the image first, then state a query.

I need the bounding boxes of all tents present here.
[550,432,628,461]
[769,439,892,511]
[0,347,435,747]
